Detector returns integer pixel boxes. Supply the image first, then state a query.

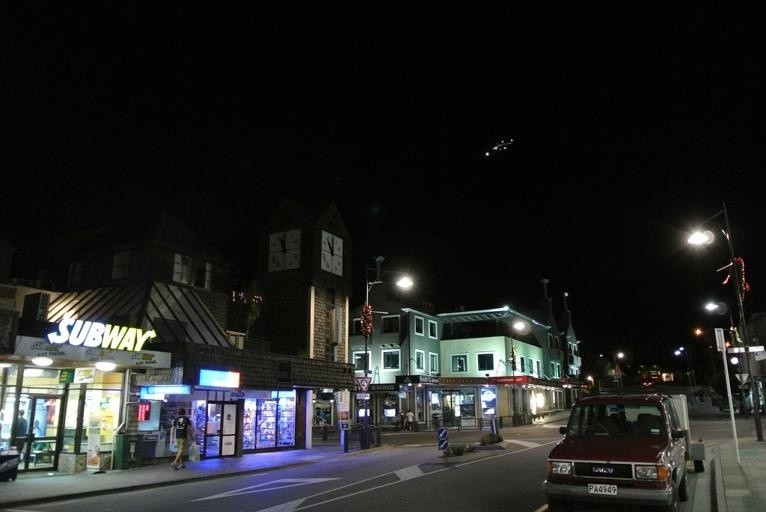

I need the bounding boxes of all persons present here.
[169,407,195,470]
[398,408,415,432]
[7,408,28,464]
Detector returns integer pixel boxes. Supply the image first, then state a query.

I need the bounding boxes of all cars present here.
[641,378,657,388]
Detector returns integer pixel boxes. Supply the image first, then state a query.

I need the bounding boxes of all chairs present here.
[584,412,666,436]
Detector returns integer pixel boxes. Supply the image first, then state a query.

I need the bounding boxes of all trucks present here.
[710,374,765,416]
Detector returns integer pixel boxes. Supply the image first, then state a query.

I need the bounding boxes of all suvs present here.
[542,392,688,511]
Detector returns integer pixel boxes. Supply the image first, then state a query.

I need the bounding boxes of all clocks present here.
[266,228,302,272]
[320,228,346,277]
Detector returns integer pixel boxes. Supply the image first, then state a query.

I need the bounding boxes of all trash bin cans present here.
[115,434,143,469]
[369,427,380,446]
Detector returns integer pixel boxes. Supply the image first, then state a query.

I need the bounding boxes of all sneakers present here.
[180,463,186,468]
[171,464,179,470]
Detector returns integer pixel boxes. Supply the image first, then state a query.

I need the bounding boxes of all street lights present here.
[509,318,525,426]
[702,300,736,346]
[688,194,763,442]
[673,344,698,391]
[364,268,417,443]
[611,350,625,376]
